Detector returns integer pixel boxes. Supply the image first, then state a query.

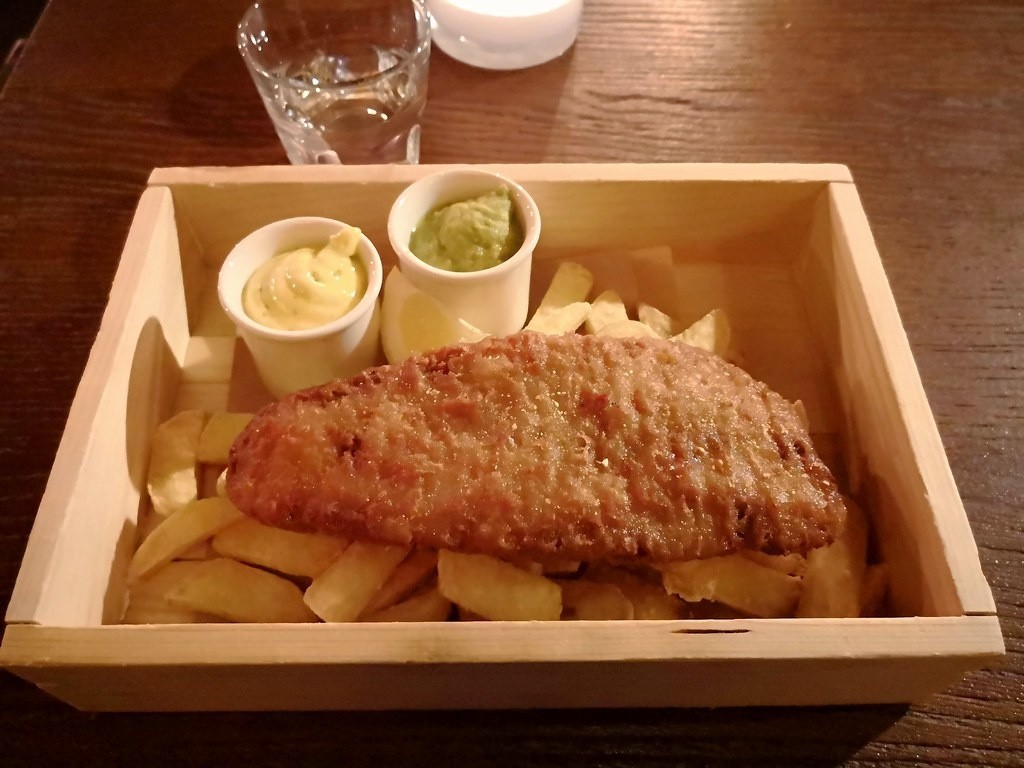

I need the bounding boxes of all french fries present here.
[123,265,888,619]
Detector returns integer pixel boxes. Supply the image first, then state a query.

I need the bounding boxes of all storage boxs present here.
[0,167,1006,709]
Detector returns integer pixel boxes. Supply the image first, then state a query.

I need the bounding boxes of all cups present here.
[236,1,430,165]
[218,216,383,402]
[387,168,542,335]
